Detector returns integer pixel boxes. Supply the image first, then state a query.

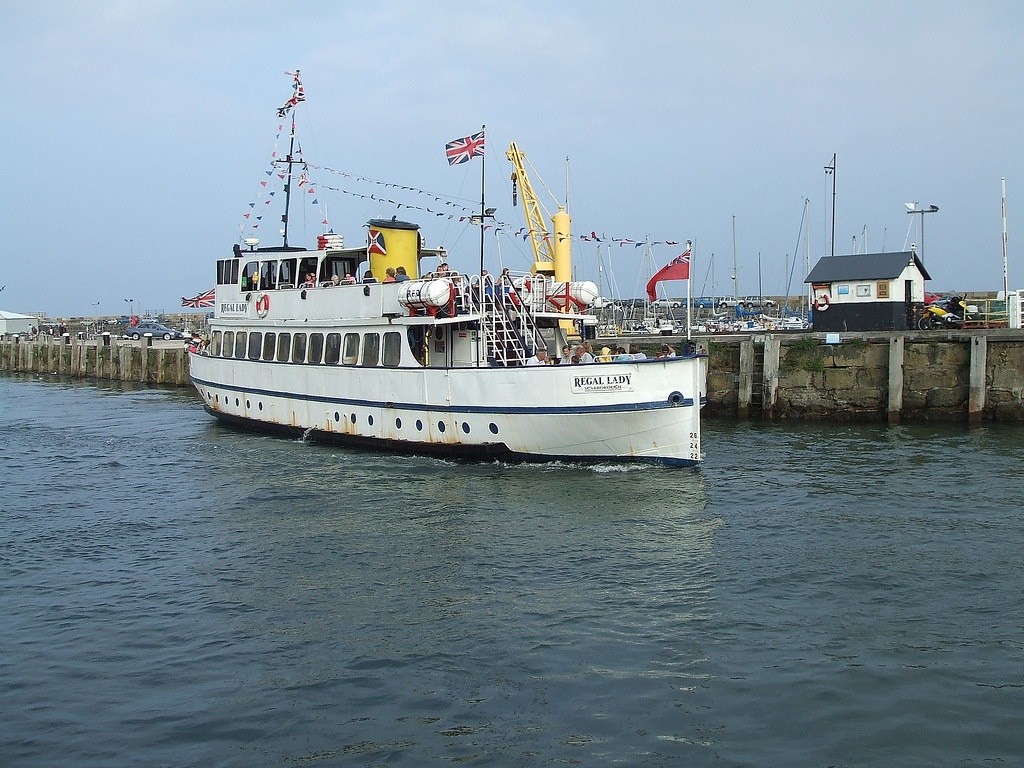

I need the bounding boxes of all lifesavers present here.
[815,294,830,312]
[255,292,270,318]
[317,235,344,250]
[509,278,532,306]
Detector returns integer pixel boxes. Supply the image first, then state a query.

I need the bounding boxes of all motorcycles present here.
[914,292,985,331]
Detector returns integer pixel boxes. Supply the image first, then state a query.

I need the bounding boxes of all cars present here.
[718,296,744,308]
[652,298,681,308]
[126,323,183,340]
[924,291,941,306]
[681,296,719,308]
[744,296,776,307]
[615,299,647,309]
[931,295,953,308]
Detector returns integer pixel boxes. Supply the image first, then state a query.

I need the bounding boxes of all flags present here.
[182,288,216,308]
[445,131,486,167]
[646,249,692,301]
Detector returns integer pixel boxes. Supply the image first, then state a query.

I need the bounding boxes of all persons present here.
[363,271,377,283]
[559,341,598,364]
[184,333,210,354]
[452,272,458,283]
[384,266,396,282]
[48,325,53,335]
[497,268,512,285]
[59,322,65,336]
[303,273,316,288]
[527,348,546,365]
[476,270,492,286]
[436,263,450,277]
[599,343,647,362]
[323,272,355,287]
[395,266,410,281]
[660,346,676,358]
[27,324,37,341]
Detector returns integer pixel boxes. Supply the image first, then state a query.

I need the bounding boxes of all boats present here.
[596,197,811,336]
[189,67,710,467]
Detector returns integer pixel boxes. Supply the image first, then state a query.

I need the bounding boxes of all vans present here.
[589,297,613,309]
